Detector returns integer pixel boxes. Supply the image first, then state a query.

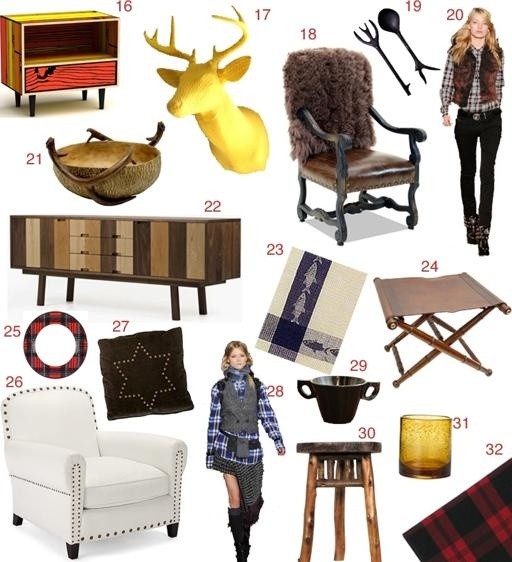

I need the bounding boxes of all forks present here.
[351,19,413,98]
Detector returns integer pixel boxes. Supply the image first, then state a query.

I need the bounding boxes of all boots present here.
[464,215,489,255]
[228,508,250,562]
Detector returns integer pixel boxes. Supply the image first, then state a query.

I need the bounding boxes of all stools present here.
[297,442,381,562]
[374,272,512,387]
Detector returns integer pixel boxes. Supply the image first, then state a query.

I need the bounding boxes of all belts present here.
[458,109,501,121]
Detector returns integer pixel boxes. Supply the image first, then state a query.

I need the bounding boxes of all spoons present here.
[378,8,438,83]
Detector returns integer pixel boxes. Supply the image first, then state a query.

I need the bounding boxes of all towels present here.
[402,457,512,562]
[255,247,367,374]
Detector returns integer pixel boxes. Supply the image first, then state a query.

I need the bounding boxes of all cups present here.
[398,413,453,482]
[298,374,382,426]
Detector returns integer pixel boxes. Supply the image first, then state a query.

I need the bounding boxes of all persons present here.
[440,7,504,257]
[206,340,285,562]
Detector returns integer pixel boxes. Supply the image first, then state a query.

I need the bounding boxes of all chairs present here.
[282,47,427,246]
[2,385,188,559]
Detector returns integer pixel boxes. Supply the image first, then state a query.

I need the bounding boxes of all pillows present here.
[98,327,193,421]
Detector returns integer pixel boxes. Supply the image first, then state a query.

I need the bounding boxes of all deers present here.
[143,4,269,174]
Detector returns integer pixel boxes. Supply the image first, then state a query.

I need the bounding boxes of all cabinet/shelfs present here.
[1,11,120,117]
[10,215,241,320]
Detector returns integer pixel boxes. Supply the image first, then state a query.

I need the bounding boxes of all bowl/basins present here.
[53,141,161,202]
